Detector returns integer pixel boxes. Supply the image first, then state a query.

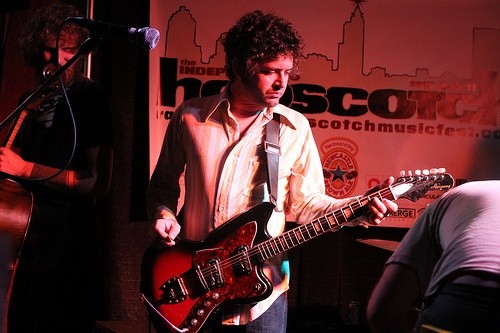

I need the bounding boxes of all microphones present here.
[70,17,161,50]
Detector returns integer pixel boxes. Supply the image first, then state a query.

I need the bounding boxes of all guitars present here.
[134,166,454,333]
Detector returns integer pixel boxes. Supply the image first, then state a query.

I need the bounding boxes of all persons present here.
[147,10,398,333]
[0,2,115,333]
[366,180,500,333]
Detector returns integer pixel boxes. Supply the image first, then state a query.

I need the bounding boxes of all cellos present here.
[0,48,66,333]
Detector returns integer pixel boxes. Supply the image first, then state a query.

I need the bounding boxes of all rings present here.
[384,210,390,217]
